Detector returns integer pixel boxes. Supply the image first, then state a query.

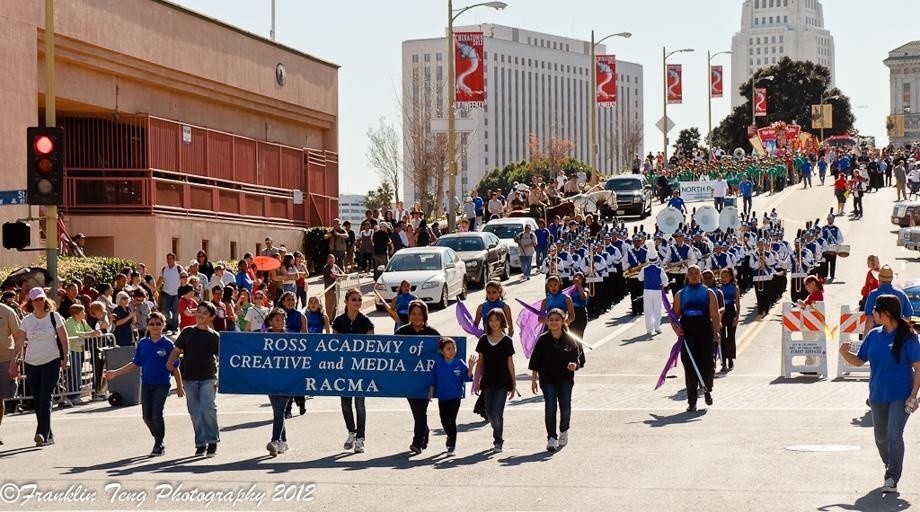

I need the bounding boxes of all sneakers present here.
[409,444,423,454]
[837,211,864,221]
[196,441,208,455]
[446,438,457,455]
[354,437,366,453]
[687,401,698,411]
[34,432,55,446]
[205,443,218,458]
[882,476,897,493]
[493,442,503,453]
[344,430,357,450]
[546,436,558,451]
[267,440,288,457]
[150,444,165,457]
[558,428,568,446]
[704,388,713,405]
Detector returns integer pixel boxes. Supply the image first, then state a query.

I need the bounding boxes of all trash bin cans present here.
[102,346,140,407]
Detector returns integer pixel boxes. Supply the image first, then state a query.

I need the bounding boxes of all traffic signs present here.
[0,189,27,205]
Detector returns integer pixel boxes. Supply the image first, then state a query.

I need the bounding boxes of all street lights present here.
[447,1,509,233]
[748,64,775,126]
[893,105,911,147]
[819,92,842,143]
[706,48,734,150]
[662,44,697,166]
[590,28,632,188]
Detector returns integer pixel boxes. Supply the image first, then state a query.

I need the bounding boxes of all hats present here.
[29,287,46,301]
[878,264,893,281]
[549,213,618,266]
[794,214,834,244]
[631,213,784,248]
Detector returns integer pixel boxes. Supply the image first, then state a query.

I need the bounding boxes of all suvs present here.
[816,134,858,155]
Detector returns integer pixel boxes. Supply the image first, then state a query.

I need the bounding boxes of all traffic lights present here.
[27,130,63,204]
[2,220,23,250]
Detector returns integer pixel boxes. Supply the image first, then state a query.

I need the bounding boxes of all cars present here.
[599,172,654,223]
[891,158,919,256]
[371,213,542,317]
